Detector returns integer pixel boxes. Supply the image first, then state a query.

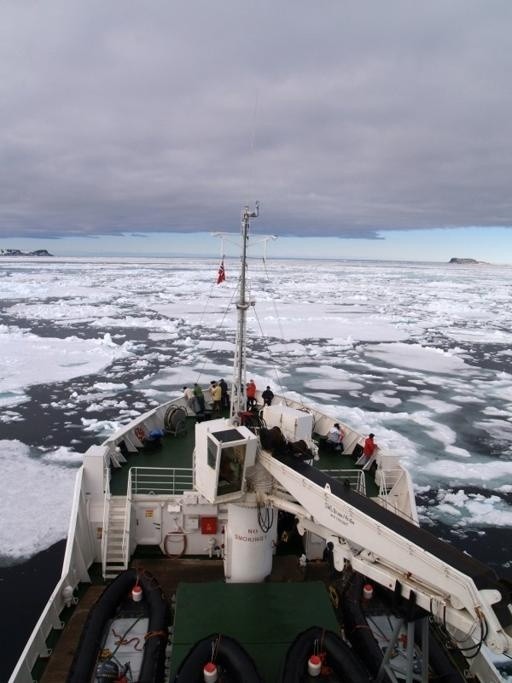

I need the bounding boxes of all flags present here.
[216,258,226,286]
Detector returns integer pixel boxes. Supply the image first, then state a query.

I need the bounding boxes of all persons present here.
[193,382,205,410]
[245,382,254,407]
[219,377,228,408]
[246,378,258,405]
[208,380,222,414]
[327,422,343,456]
[261,385,275,406]
[182,385,197,413]
[363,432,379,467]
